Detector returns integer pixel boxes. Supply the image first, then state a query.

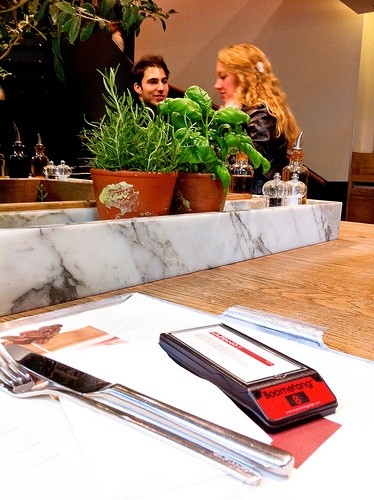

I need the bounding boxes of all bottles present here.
[262,172,292,208]
[225,150,254,200]
[8,140,31,178]
[0,153,5,178]
[286,172,307,205]
[57,160,72,181]
[281,148,309,205]
[31,144,49,178]
[43,160,58,180]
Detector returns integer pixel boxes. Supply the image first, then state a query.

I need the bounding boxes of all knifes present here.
[4,343,295,475]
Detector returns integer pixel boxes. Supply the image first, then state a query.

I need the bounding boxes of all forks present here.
[0,347,262,487]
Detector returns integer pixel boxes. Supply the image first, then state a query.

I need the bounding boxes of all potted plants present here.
[77,63,209,221]
[155,85,271,214]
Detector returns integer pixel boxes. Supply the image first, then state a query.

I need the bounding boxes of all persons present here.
[213,43,304,195]
[131,54,172,127]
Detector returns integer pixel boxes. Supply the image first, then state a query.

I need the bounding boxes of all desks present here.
[0,219,374,500]
